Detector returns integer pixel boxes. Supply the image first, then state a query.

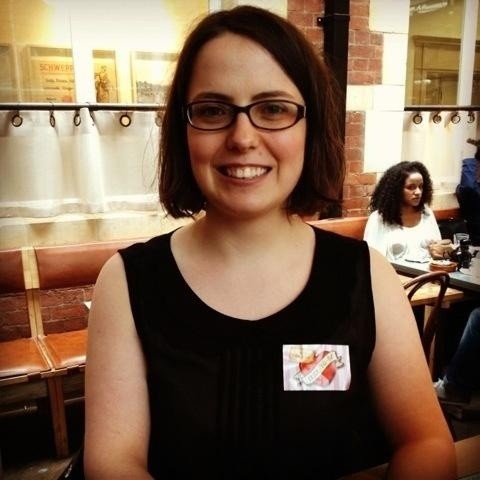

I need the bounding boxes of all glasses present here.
[183,99,306,130]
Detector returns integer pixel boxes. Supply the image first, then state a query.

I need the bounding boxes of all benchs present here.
[0,207,461,460]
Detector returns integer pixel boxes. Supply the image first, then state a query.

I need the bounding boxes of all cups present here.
[453,232,469,249]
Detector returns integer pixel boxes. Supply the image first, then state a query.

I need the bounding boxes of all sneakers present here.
[433,379,471,406]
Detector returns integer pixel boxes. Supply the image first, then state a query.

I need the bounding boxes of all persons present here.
[362,140,480,407]
[83,6,458,480]
